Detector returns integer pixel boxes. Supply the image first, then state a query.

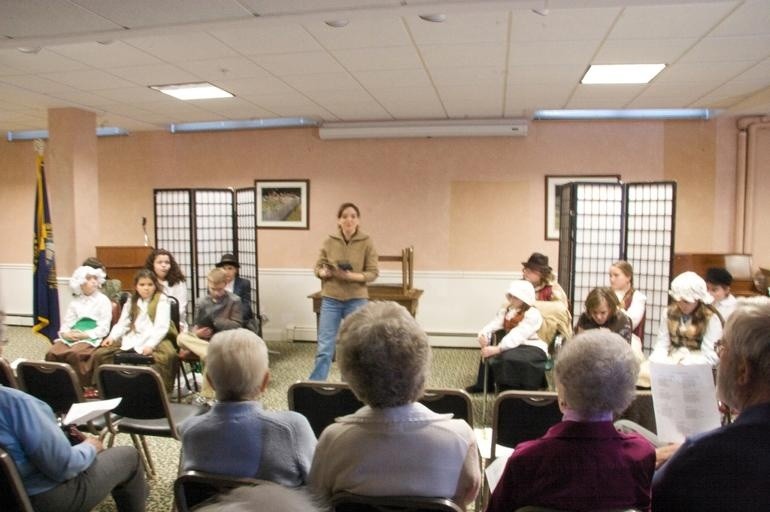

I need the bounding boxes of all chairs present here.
[618,391,656,435]
[286,384,365,439]
[0,357,16,391]
[175,470,263,511]
[418,386,474,428]
[16,362,122,454]
[325,488,460,511]
[0,450,34,511]
[487,390,560,458]
[93,364,207,476]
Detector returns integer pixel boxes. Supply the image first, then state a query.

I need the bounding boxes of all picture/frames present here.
[545,175,621,240]
[254,180,310,229]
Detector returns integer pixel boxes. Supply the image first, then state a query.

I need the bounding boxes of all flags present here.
[31,150,60,344]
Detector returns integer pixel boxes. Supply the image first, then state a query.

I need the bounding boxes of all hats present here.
[215,254,239,267]
[522,253,553,273]
[504,280,537,307]
[707,267,732,286]
[69,266,107,296]
[668,271,715,305]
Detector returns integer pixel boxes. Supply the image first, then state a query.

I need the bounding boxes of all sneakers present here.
[466,384,495,393]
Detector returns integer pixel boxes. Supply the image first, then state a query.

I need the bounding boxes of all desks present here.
[307,288,423,362]
[306,246,423,300]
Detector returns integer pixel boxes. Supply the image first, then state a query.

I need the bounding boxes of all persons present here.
[573,261,649,366]
[648,266,736,368]
[177,326,317,487]
[47,250,251,401]
[652,296,770,505]
[307,202,378,382]
[0,366,148,512]
[481,327,648,512]
[307,302,478,512]
[192,487,328,512]
[466,253,573,396]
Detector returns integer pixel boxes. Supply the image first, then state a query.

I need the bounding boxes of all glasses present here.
[713,340,728,356]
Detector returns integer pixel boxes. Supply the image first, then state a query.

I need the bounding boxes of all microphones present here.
[142,217,150,247]
